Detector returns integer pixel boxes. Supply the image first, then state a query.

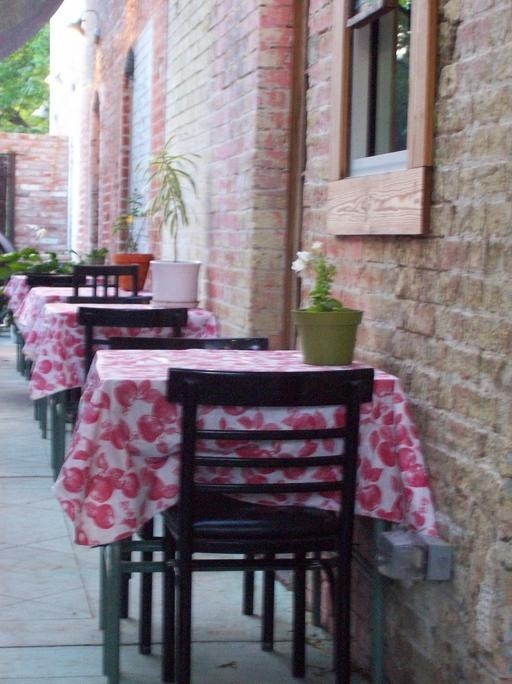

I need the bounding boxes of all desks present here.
[8,277,217,479]
[79,345,403,681]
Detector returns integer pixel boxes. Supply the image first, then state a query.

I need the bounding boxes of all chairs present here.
[161,366,372,681]
[67,261,269,350]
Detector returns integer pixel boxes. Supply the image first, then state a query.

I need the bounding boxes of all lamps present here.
[67,6,105,46]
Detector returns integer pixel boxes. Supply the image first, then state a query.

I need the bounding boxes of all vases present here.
[286,306,364,366]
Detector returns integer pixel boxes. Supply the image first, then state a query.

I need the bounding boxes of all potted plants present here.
[110,181,155,290]
[137,132,206,308]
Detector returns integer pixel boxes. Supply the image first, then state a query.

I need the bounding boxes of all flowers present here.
[289,242,343,311]
